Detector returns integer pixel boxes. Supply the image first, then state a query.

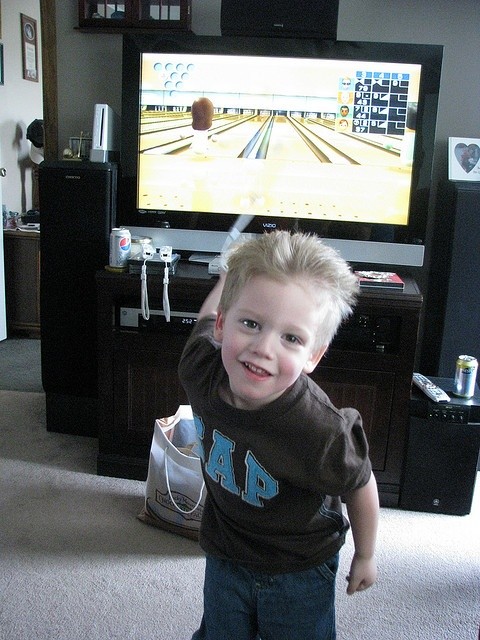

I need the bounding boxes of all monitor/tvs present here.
[115,32,444,264]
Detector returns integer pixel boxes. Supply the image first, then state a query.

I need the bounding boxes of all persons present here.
[177,230,380,639]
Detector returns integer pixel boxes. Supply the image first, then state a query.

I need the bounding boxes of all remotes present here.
[412,372,451,404]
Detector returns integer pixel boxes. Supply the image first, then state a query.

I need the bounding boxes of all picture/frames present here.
[20,12,39,83]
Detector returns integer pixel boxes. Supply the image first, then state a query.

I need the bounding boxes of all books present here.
[128,252,180,275]
[354,268,404,293]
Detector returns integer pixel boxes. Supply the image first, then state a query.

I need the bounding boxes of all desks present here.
[3,224,41,337]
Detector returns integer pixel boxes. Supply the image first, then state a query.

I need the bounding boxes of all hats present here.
[26,119,43,148]
[30,143,44,164]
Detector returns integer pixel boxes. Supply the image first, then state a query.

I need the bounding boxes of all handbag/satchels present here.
[136,405,208,541]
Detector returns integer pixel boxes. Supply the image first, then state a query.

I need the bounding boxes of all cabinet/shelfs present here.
[98,264,423,509]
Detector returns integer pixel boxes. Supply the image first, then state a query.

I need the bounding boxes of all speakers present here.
[398,376,480,516]
[37,158,114,439]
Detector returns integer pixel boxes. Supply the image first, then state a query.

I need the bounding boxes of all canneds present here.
[451,354,478,400]
[108,227,132,269]
